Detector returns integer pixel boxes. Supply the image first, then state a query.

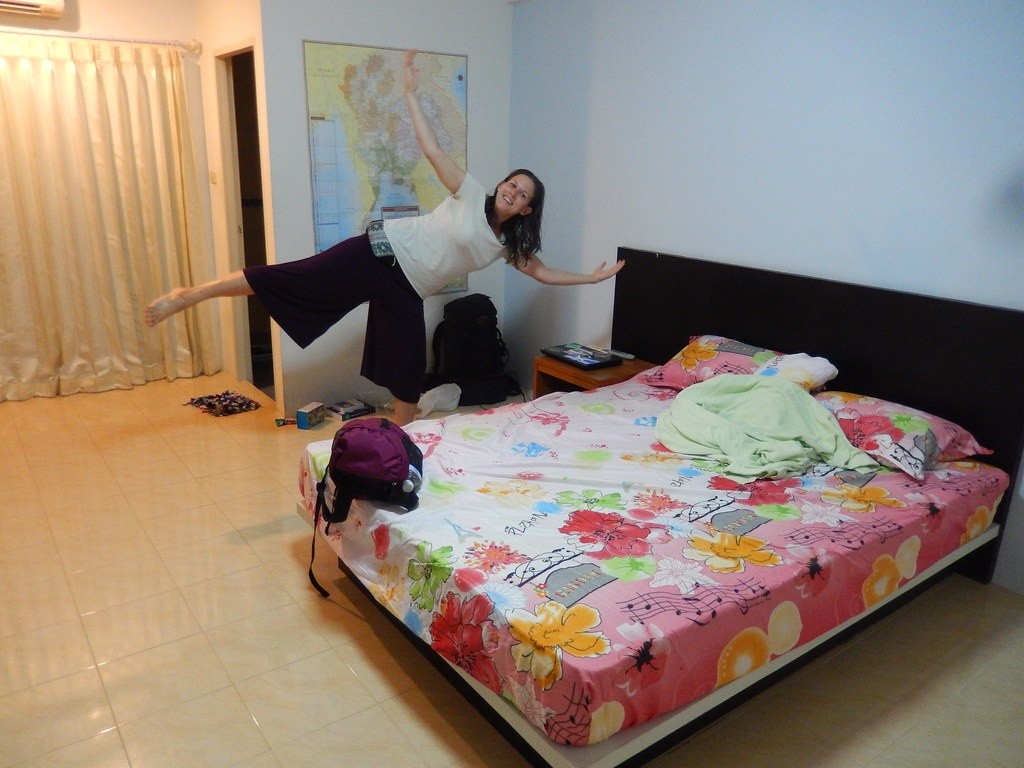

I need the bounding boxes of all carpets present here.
[181,389,261,418]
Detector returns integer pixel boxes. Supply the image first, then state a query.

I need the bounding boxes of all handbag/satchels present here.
[366,219,394,257]
[316,418,423,523]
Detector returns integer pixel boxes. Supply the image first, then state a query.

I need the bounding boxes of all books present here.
[327,399,375,421]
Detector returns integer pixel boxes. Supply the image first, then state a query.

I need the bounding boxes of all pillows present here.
[648,333,835,400]
[809,387,993,482]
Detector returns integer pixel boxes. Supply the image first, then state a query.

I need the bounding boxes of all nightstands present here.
[531,345,657,398]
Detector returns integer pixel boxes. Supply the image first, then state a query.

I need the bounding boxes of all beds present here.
[298,247,1024,768]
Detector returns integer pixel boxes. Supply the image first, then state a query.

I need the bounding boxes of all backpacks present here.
[432,294,522,406]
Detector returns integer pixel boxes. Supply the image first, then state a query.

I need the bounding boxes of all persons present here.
[144,50,625,425]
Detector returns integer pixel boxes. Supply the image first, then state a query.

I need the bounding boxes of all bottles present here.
[402,459,423,493]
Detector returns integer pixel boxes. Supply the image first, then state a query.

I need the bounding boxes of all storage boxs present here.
[297,401,324,430]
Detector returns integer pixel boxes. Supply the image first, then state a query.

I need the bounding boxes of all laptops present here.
[540,343,624,371]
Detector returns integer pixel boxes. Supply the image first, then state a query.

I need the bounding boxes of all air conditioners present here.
[0,0,66,20]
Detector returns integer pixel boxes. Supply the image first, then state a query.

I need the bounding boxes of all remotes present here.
[608,350,636,360]
